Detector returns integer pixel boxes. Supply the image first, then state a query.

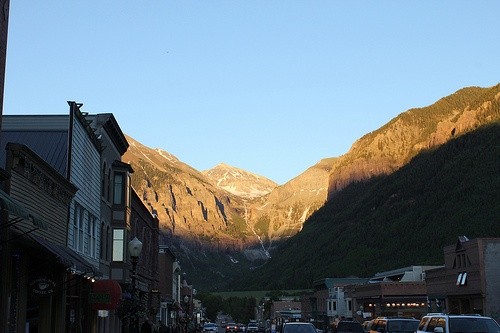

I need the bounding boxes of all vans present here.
[203,323,216,333]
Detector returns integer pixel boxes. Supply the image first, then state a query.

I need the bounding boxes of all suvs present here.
[226,323,238,332]
[368,316,421,333]
[416,312,500,333]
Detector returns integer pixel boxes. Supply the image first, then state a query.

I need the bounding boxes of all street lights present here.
[183,295,190,333]
[127,236,143,332]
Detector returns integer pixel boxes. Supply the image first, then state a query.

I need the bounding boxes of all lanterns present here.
[89,280,123,312]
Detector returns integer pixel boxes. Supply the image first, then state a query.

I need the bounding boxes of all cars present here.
[216,312,231,328]
[247,323,258,332]
[333,321,366,333]
[215,324,219,332]
[281,322,319,333]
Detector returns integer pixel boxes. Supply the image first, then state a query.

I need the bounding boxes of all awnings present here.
[0,189,48,248]
[12,225,103,295]
